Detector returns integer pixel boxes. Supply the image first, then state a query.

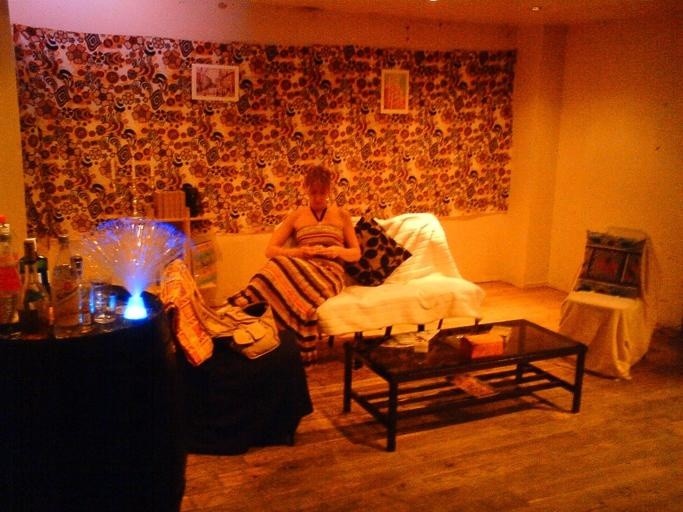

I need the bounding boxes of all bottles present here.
[0,213,86,336]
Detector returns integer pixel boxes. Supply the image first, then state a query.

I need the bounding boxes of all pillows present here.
[573,229,648,299]
[341,208,412,287]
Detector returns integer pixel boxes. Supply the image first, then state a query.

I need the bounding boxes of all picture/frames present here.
[190,63,240,102]
[380,70,410,116]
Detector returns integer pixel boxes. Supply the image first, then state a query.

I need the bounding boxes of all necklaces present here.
[310,206,327,224]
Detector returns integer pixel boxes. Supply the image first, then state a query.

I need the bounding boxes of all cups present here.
[88,288,118,324]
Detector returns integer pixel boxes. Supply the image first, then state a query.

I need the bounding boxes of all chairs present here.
[165,257,297,445]
[556,226,656,382]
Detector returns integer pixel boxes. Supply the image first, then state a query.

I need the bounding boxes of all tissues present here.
[415,329,440,354]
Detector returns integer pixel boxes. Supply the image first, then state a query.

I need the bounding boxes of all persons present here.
[218,164,362,371]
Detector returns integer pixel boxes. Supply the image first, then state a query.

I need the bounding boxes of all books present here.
[451,370,500,399]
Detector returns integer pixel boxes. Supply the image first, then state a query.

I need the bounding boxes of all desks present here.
[0,281,164,512]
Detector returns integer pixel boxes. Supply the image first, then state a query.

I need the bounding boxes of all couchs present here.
[212,212,483,370]
